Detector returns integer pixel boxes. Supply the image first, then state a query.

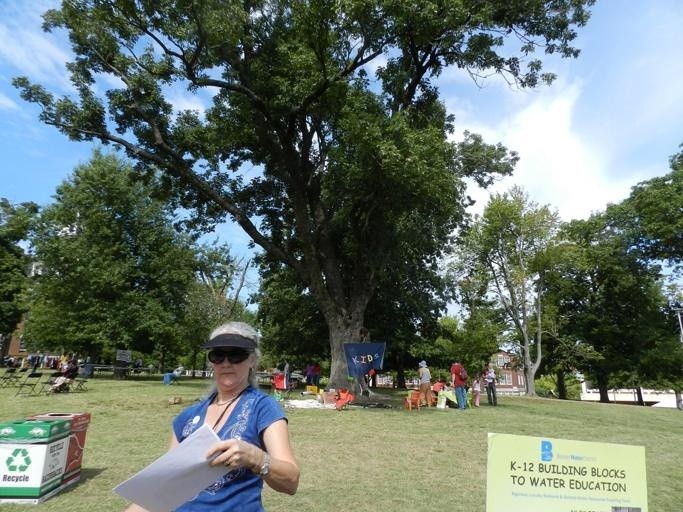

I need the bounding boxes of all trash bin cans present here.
[254,450,272,478]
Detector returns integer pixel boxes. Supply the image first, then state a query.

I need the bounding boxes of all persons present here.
[273,358,322,391]
[163,363,185,387]
[48,352,85,395]
[123,321,300,512]
[417,358,498,410]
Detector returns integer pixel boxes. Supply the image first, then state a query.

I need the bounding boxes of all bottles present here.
[419,360,427,367]
[204,333,257,350]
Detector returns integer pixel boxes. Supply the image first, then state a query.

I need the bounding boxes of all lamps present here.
[0,363,94,396]
[403,392,421,411]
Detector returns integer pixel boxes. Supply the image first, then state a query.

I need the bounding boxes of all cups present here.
[208,349,249,364]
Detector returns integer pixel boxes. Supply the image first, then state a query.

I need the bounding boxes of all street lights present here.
[459,367,468,380]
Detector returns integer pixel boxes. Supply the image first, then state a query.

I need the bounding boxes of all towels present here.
[0,413,90,504]
